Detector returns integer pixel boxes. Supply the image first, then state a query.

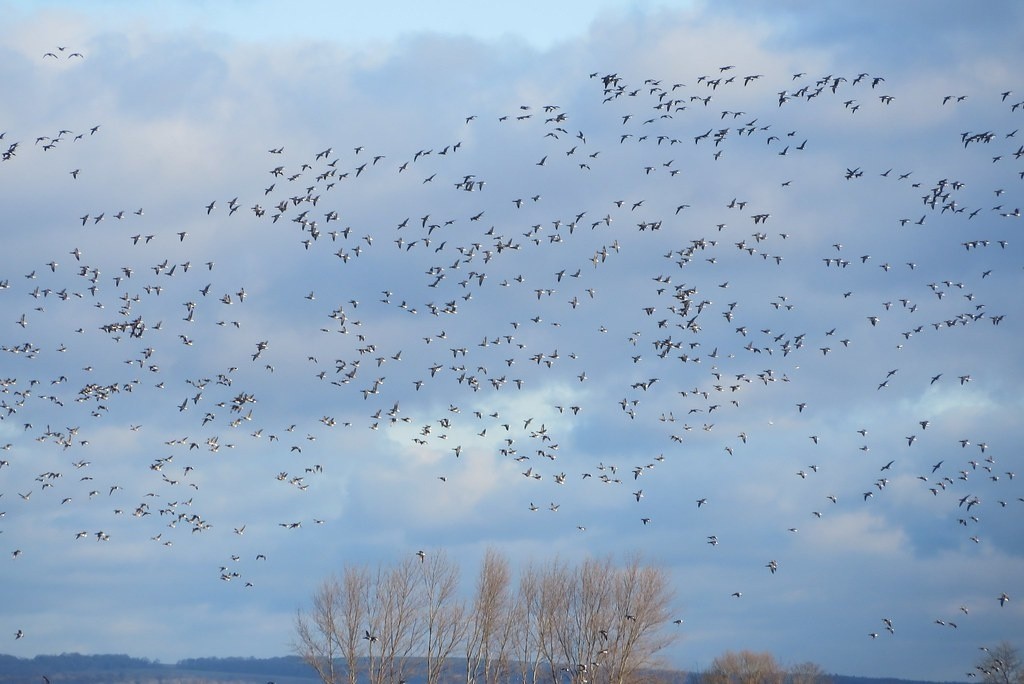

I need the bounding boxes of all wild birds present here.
[0,37,1023,669]
